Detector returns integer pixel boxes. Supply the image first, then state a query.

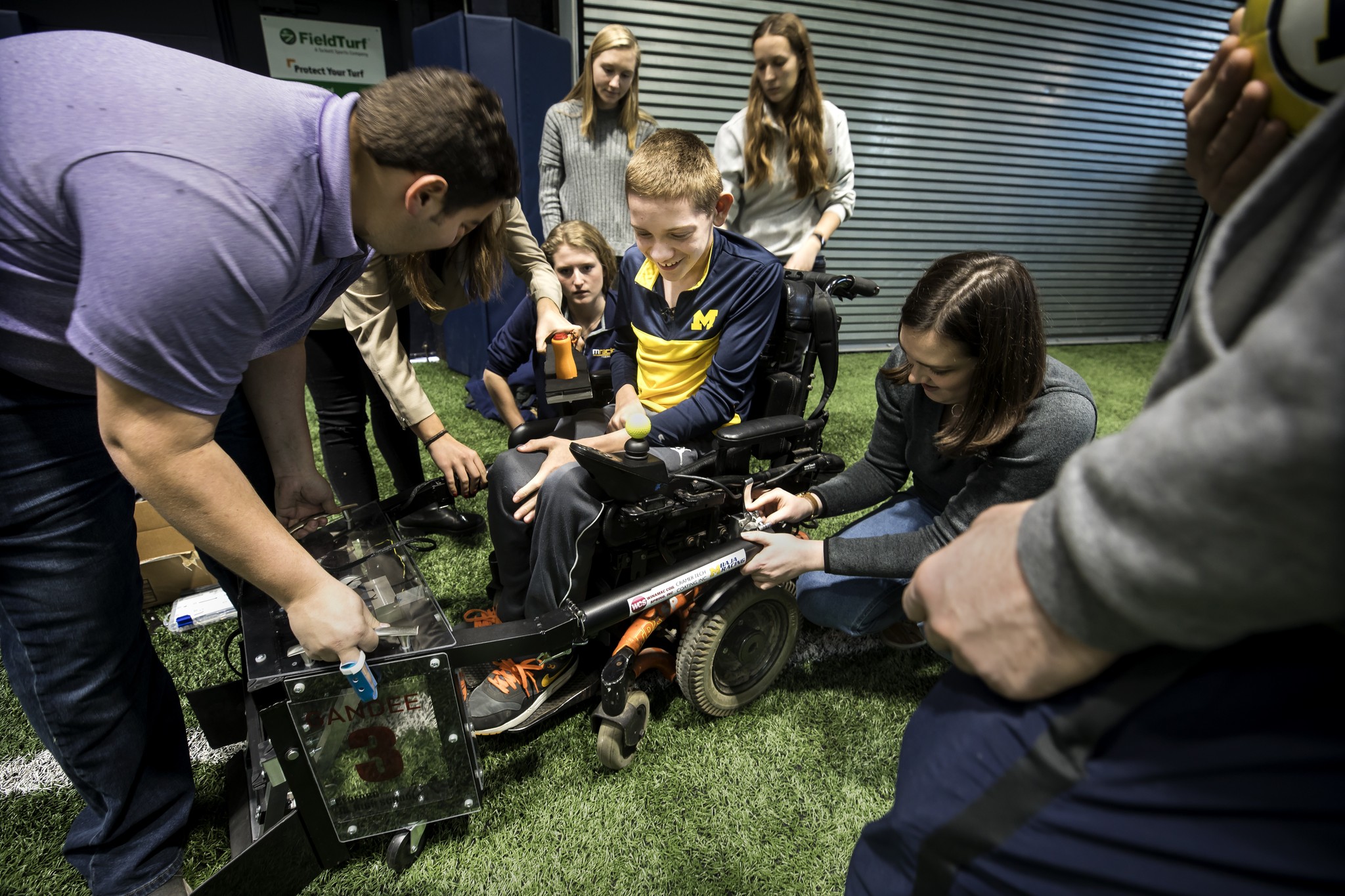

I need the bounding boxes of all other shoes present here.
[881,617,927,648]
[144,868,193,896]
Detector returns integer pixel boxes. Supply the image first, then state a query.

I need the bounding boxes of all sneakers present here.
[450,602,504,628]
[466,649,577,735]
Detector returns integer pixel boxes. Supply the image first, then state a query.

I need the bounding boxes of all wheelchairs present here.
[476,250,882,732]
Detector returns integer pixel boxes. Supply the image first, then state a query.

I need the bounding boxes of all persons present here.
[714,13,855,380]
[452,128,786,735]
[741,251,1098,648]
[0,31,518,896]
[538,24,659,270]
[845,0,1345,896]
[484,220,617,430]
[305,197,586,537]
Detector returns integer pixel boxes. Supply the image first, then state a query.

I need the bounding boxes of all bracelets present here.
[812,233,826,251]
[423,429,449,449]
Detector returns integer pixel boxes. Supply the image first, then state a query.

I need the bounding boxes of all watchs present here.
[794,491,819,520]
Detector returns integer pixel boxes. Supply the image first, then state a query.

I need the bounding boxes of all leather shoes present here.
[398,501,487,538]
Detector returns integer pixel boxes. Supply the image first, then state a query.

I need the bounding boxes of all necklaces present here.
[951,403,964,417]
